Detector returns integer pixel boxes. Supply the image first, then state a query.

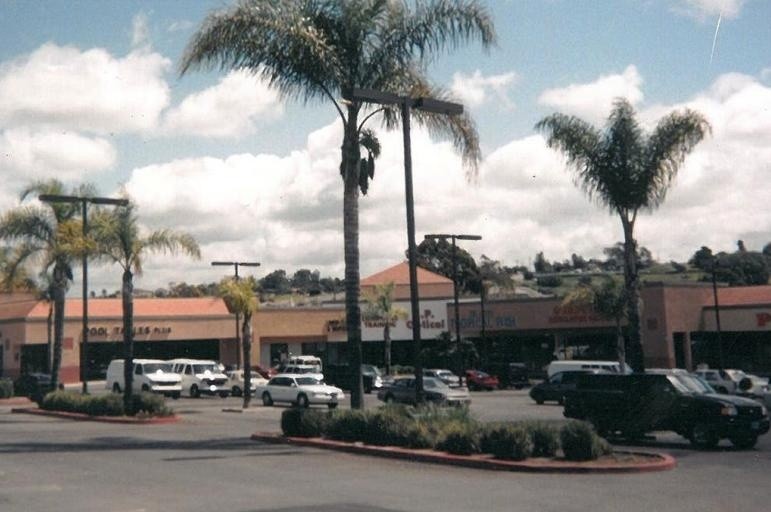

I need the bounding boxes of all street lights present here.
[339,82,466,403]
[422,233,484,386]
[36,191,132,396]
[209,258,265,412]
[696,257,733,368]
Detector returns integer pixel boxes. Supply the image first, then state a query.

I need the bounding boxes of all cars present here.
[425,368,466,386]
[16,372,65,400]
[285,355,376,393]
[464,369,497,391]
[256,373,345,409]
[529,360,771,450]
[228,370,268,397]
[377,374,471,405]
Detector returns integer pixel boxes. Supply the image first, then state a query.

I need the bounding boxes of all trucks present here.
[106,357,184,400]
[172,356,232,397]
